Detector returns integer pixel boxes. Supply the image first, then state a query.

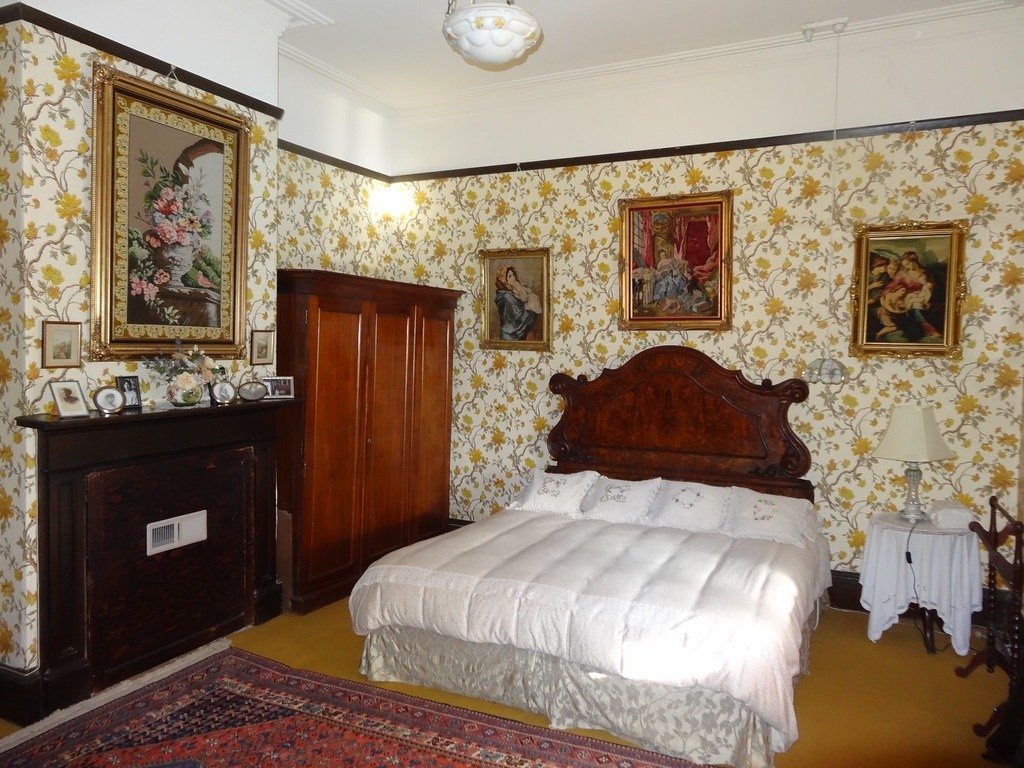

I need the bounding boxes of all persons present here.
[123,380,138,406]
[278,379,288,395]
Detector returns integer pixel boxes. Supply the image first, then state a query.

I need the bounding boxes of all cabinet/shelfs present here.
[14,394,308,710]
[277,269,466,616]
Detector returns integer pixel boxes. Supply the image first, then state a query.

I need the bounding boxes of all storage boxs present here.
[933,500,973,529]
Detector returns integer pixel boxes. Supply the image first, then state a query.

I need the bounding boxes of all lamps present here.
[442,0,541,65]
[871,406,954,523]
[801,32,849,387]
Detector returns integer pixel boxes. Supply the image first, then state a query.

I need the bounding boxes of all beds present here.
[365,345,828,758]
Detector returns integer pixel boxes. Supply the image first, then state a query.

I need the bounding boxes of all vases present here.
[167,385,203,407]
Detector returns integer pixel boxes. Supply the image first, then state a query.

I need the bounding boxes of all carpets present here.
[0,637,723,768]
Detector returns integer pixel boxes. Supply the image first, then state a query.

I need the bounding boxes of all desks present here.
[873,511,977,653]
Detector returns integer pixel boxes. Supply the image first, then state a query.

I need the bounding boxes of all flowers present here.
[141,336,221,390]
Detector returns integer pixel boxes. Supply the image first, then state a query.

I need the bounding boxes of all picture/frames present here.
[48,376,143,418]
[211,376,294,403]
[478,247,553,351]
[41,321,82,369]
[848,218,969,360]
[251,330,274,365]
[89,61,253,362]
[618,189,733,331]
